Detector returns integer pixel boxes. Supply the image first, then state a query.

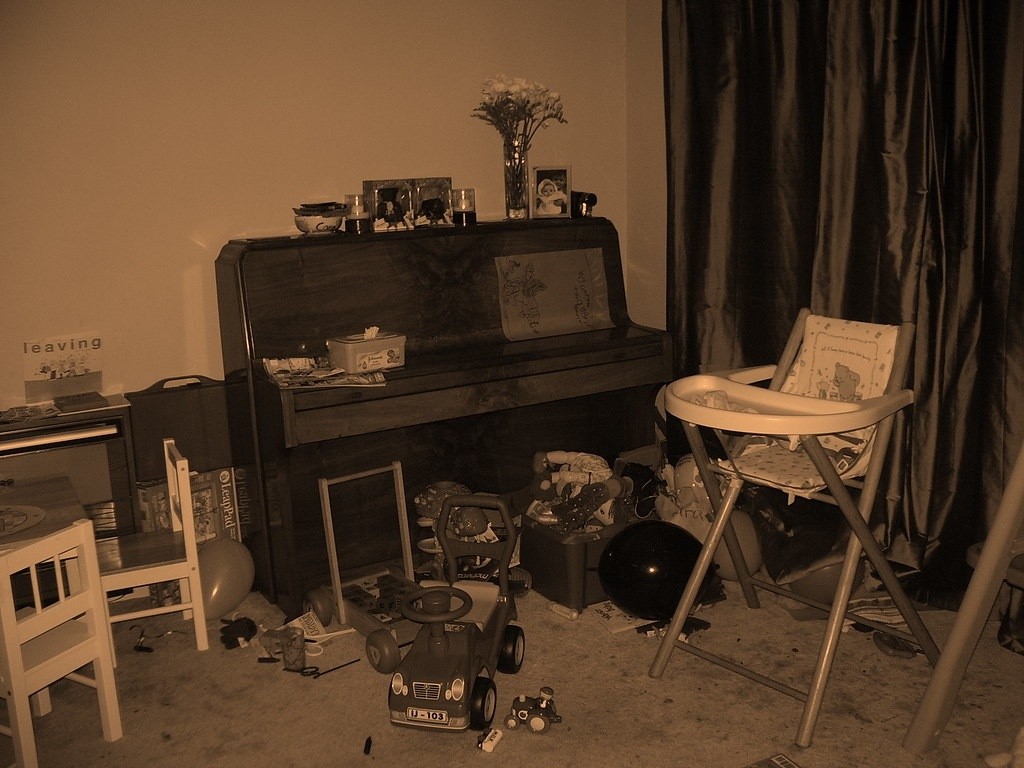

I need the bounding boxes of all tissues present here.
[324,325,407,375]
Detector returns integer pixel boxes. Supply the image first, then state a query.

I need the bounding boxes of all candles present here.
[459,190,471,208]
[351,196,364,213]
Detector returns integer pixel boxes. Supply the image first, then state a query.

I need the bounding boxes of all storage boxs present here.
[519,505,656,613]
[996,554,1024,655]
[363,177,456,234]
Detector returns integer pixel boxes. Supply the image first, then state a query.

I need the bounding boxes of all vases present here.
[503,141,528,219]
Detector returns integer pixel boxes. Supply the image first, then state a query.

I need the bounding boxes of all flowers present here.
[467,69,570,211]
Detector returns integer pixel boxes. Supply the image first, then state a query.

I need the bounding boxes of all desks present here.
[1,477,94,673]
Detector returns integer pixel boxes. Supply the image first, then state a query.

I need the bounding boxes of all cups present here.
[279,627,305,672]
[344,194,365,215]
[451,188,475,212]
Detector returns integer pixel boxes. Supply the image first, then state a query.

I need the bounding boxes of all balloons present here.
[599,520,715,619]
[197,539,255,620]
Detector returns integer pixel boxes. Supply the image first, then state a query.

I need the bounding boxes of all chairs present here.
[96,437,210,668]
[644,309,940,748]
[0,518,123,768]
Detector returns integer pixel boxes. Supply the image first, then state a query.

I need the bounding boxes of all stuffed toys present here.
[536,463,661,533]
[657,452,762,580]
[533,451,630,526]
[415,482,488,537]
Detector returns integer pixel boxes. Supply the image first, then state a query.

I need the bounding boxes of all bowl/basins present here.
[294,215,342,234]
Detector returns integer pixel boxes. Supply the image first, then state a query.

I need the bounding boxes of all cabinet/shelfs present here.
[0,408,151,603]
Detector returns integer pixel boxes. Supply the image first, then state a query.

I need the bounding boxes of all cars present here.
[388,493,525,731]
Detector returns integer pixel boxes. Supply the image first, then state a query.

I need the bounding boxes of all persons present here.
[537,170,567,215]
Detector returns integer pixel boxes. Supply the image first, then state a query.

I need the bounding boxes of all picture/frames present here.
[531,165,573,218]
